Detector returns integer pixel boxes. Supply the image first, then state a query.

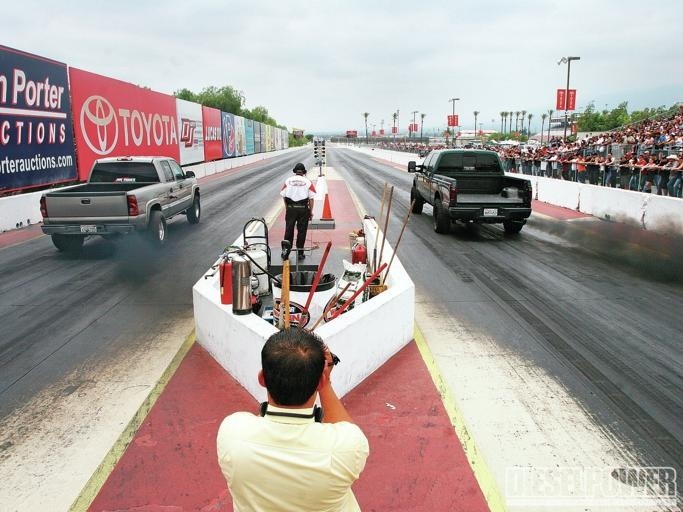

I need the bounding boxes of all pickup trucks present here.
[405,148,532,235]
[40,154,200,252]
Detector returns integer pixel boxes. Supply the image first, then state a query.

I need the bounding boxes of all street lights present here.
[448,98,461,144]
[556,56,579,141]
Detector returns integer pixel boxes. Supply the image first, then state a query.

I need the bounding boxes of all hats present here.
[295,163,305,170]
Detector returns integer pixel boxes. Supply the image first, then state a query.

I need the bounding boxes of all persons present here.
[279,163,314,261]
[216,328,370,512]
[413,102,683,198]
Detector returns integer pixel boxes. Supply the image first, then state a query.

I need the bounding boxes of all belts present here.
[288,205,305,208]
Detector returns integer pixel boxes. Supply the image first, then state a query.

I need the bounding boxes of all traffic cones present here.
[319,193,334,220]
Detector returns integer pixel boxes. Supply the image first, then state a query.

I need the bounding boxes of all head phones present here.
[293,163,307,174]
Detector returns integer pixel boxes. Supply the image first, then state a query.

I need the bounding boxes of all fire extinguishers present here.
[232,250,253,314]
[219,255,235,304]
